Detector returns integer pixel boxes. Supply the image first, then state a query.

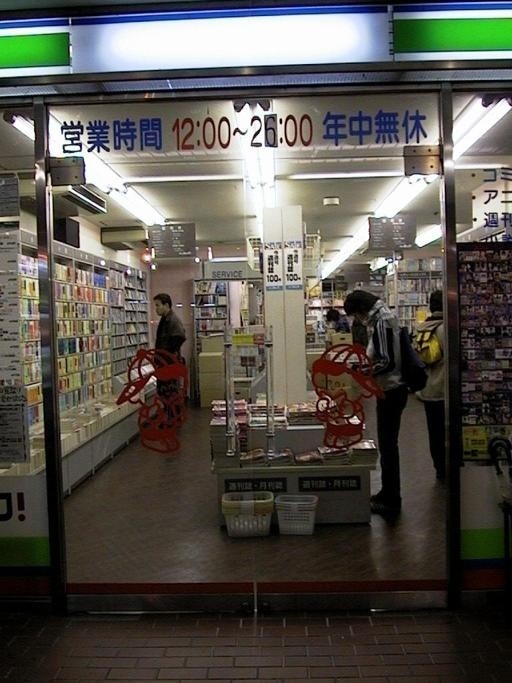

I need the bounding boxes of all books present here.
[191,277,267,408]
[311,247,442,369]
[5,251,155,480]
[211,391,379,467]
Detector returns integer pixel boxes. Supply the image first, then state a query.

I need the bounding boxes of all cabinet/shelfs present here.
[190,277,265,407]
[0,229,158,513]
[205,397,379,524]
[306,234,443,352]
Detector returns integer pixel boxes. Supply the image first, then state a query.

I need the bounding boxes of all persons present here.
[412,289,447,478]
[153,292,188,430]
[344,290,407,520]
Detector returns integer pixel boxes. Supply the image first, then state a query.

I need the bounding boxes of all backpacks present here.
[414,319,444,368]
[399,326,428,384]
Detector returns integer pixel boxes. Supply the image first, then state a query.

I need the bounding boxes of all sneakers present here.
[370,490,400,505]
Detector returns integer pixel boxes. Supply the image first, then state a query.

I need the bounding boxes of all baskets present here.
[221,490,319,536]
